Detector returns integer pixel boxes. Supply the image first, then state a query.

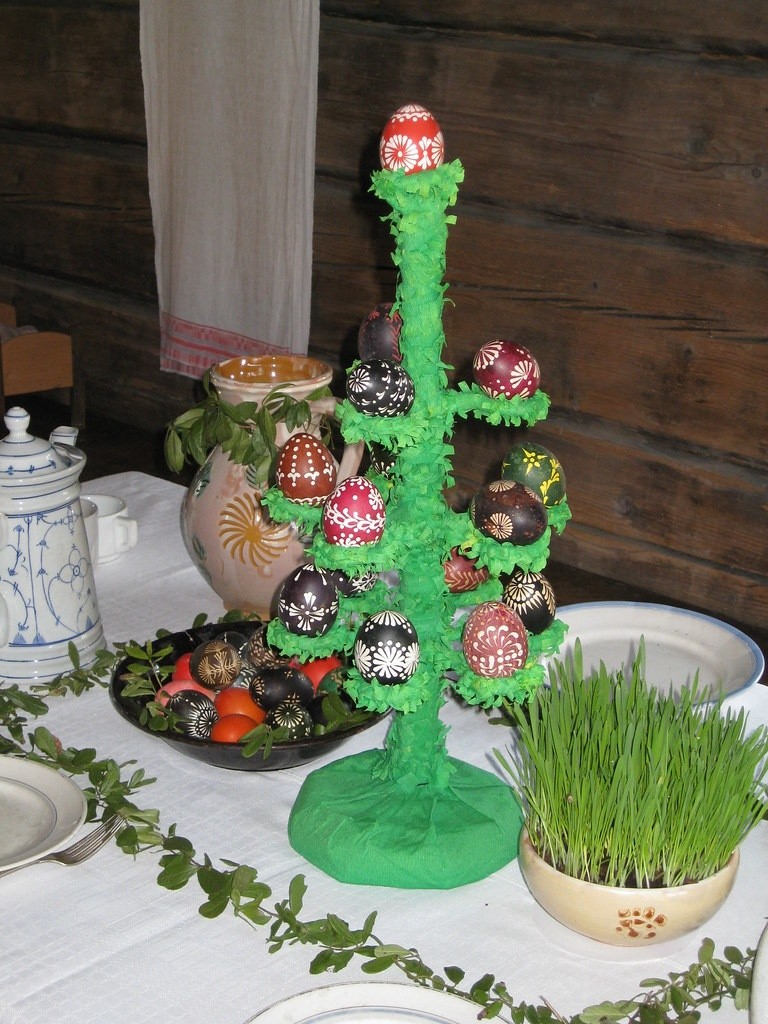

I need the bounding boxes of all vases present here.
[180,353,365,619]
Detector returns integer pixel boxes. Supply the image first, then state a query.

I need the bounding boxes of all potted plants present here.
[494,632,768,946]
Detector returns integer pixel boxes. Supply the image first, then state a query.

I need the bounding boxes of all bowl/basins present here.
[108,620,393,774]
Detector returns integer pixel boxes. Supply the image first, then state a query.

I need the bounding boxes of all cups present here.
[77,499,99,571]
[80,495,137,564]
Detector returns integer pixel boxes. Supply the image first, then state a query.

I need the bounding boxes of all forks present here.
[0,814,126,879]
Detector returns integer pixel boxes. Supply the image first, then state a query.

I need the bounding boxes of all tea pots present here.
[0,407,110,698]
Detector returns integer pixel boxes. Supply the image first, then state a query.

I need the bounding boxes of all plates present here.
[0,753,88,878]
[243,982,513,1024]
[535,601,765,708]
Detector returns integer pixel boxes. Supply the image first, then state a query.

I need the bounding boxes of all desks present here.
[0,471,768,1024]
